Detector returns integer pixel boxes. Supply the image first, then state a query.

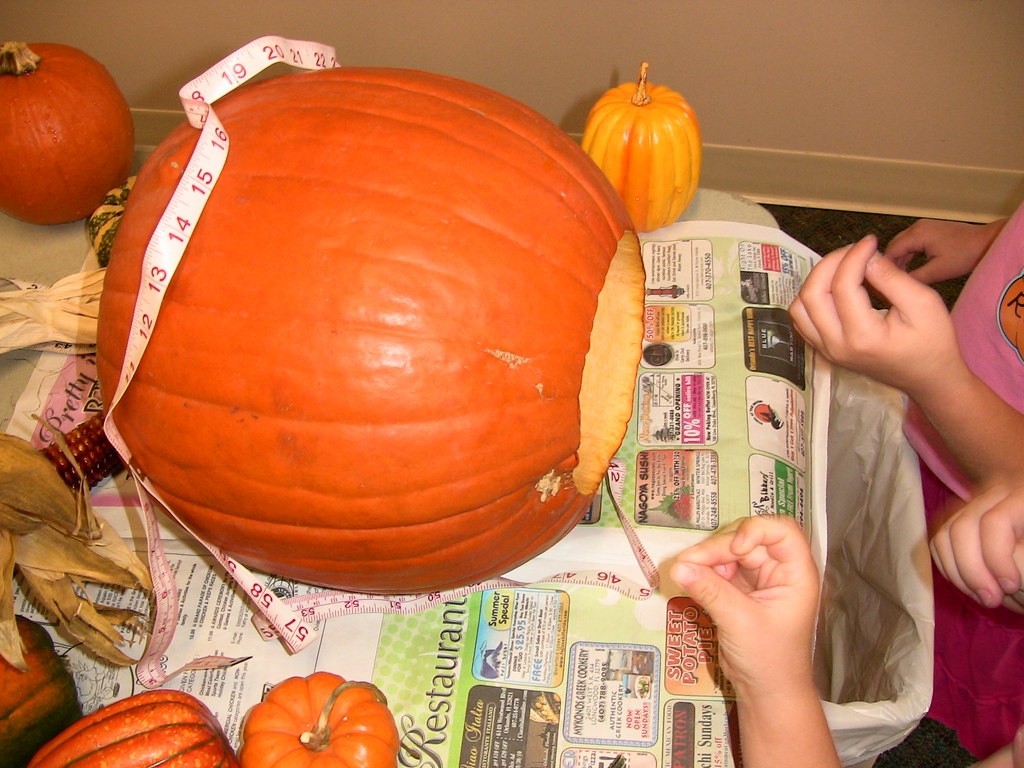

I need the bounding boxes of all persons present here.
[788,197,1024,768]
[667,513,844,768]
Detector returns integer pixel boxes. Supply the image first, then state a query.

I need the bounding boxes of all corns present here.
[40,409,117,497]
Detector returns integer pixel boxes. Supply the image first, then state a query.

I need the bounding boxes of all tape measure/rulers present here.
[103,34,661,688]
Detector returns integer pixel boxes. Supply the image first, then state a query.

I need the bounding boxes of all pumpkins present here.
[0,42,135,225]
[581,63,702,233]
[0,616,398,768]
[89,176,138,266]
